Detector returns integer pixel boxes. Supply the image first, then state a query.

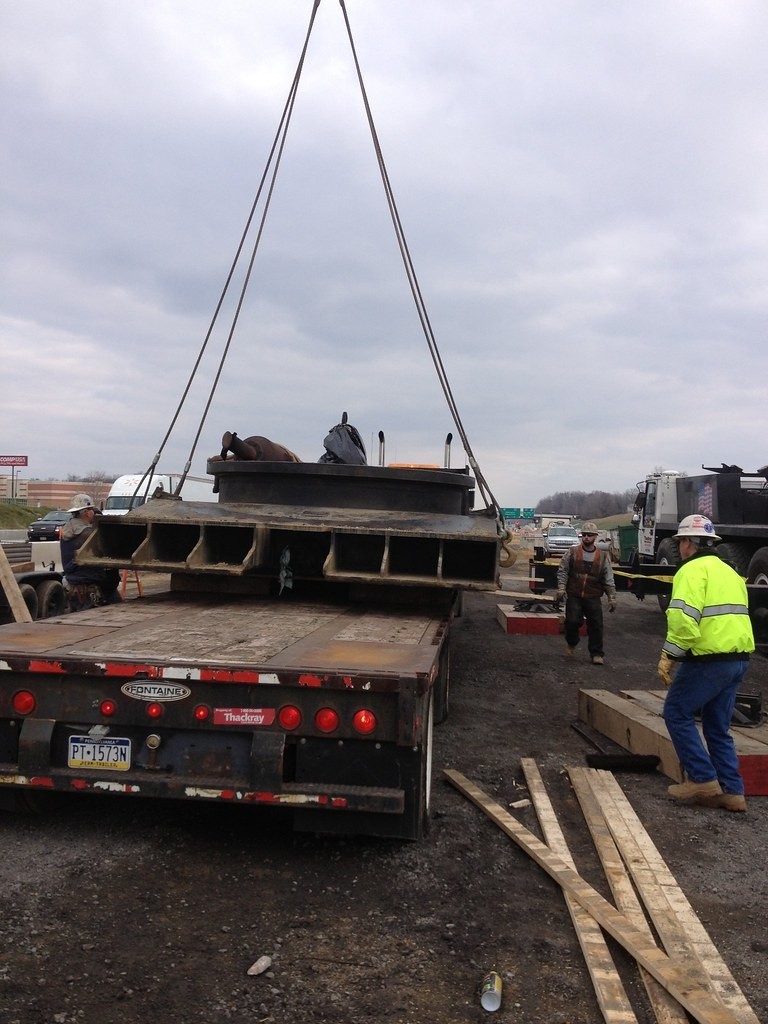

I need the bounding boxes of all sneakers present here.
[698,794,746,811]
[668,780,722,798]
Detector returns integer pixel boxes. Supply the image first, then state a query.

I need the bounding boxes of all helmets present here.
[580,523,599,535]
[673,514,722,541]
[66,494,95,512]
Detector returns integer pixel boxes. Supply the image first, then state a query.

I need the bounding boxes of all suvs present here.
[542,525,583,558]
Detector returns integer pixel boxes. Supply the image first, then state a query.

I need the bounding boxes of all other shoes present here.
[592,656,604,665]
[567,644,577,657]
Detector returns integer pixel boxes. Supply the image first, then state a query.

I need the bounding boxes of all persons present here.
[557,520,617,665]
[60,494,115,612]
[659,515,757,812]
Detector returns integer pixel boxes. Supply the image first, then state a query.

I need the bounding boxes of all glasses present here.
[582,534,594,537]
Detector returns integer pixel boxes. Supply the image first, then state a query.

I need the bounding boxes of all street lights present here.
[14,470,22,497]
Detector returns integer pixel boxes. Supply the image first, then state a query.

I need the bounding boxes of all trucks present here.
[630,471,768,657]
[0,432,471,844]
[99,474,219,515]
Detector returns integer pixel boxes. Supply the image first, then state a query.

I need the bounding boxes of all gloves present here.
[607,595,616,613]
[555,589,568,602]
[657,652,675,686]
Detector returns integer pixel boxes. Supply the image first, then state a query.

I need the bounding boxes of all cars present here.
[27,509,73,541]
